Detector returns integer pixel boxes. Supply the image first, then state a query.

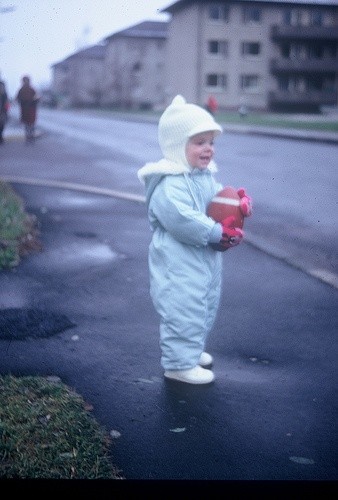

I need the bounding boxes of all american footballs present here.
[207,186,245,252]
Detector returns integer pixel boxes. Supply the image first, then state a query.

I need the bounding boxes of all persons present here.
[135,95,253,385]
[0,81,10,145]
[17,77,39,138]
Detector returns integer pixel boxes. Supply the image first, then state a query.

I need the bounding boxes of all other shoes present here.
[197,350,214,366]
[164,363,215,385]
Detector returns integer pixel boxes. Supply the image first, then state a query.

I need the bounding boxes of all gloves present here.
[237,188,253,217]
[216,216,243,250]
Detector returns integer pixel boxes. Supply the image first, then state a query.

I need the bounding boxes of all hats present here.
[158,94,223,171]
